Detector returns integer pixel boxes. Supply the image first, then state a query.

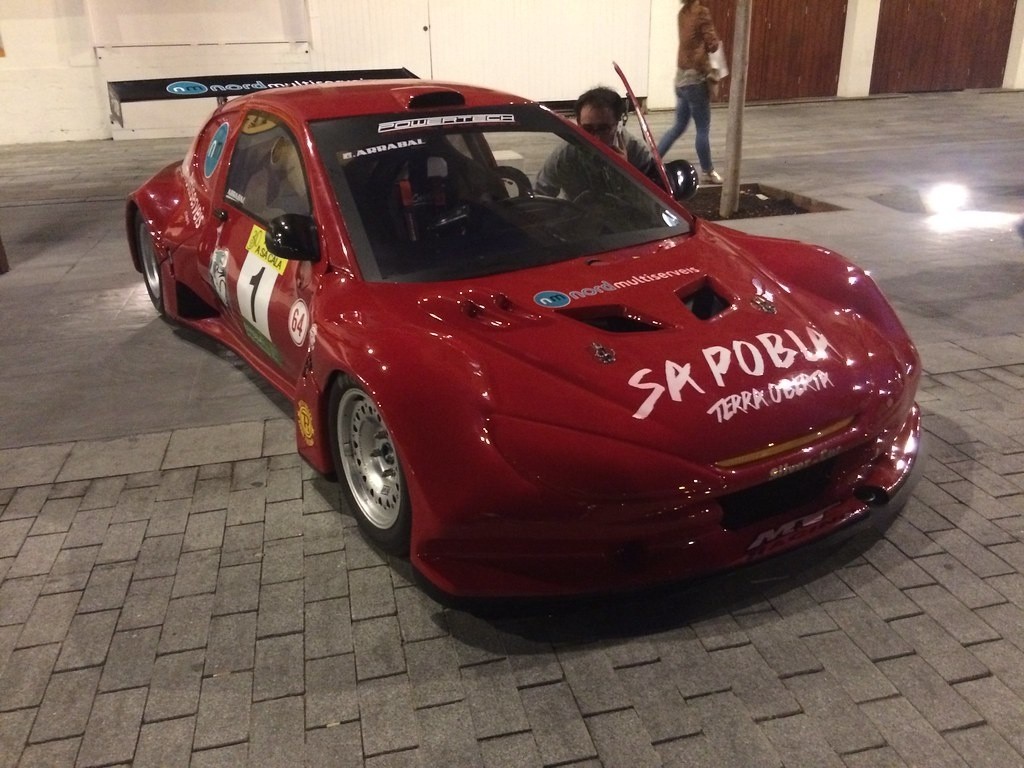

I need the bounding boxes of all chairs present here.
[386,149,476,261]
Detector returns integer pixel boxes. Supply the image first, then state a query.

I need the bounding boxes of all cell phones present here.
[613,120,625,147]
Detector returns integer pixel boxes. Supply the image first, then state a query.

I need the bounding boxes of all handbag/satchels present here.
[707,41,729,84]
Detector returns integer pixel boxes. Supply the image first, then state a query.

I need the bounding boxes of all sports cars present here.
[110,64,923,600]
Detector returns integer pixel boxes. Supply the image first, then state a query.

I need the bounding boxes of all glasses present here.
[575,120,618,137]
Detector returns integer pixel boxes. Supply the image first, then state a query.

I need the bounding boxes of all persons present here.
[516,88,667,242]
[658,0,724,183]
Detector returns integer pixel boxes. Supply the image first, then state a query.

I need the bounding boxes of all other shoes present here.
[700,170,725,184]
